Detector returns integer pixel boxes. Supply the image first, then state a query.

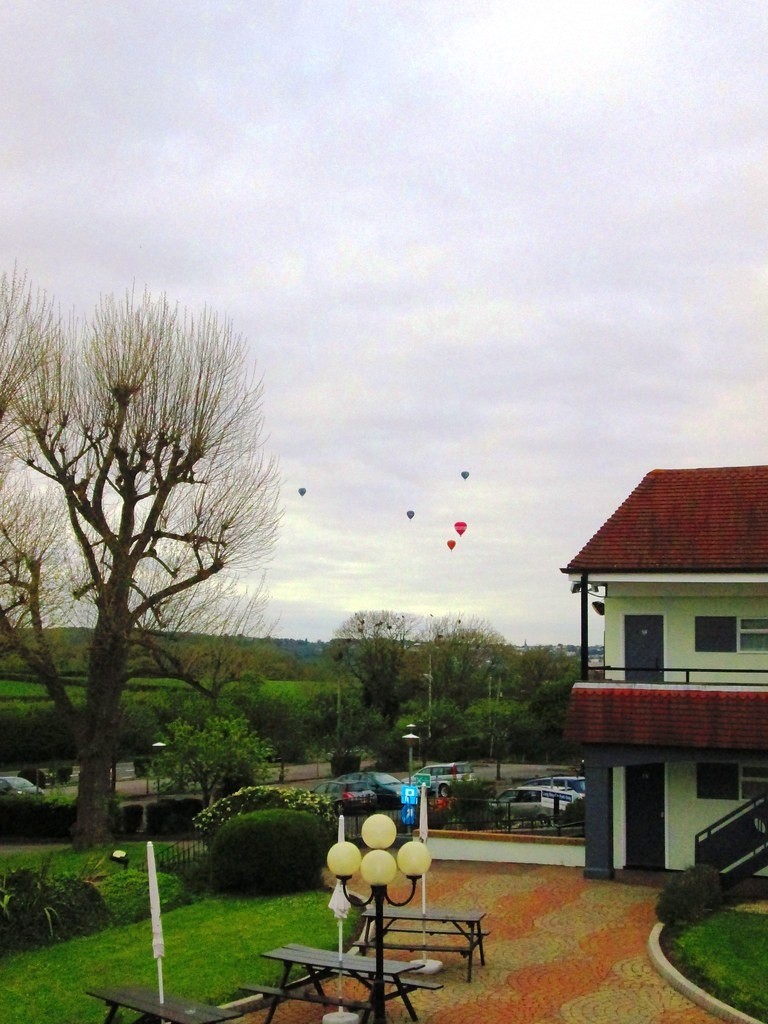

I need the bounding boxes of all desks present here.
[361,907,486,983]
[85,985,242,1024]
[260,943,425,1024]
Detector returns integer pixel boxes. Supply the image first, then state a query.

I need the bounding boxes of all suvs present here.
[336,772,408,808]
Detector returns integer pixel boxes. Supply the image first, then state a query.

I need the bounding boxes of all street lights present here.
[327,813,431,1024]
[402,724,420,784]
[153,742,167,801]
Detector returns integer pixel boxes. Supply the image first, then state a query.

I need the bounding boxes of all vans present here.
[524,776,586,795]
[490,786,581,815]
[401,761,475,798]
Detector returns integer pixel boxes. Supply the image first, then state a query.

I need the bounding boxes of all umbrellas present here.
[147,841,164,1004]
[327,815,352,1017]
[419,783,427,964]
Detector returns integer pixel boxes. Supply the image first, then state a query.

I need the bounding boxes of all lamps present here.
[110,850,129,869]
[570,581,581,594]
[592,601,604,615]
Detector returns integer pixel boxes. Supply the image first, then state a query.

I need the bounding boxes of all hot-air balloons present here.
[461,471,469,480]
[298,488,306,497]
[447,540,456,551]
[407,511,414,520]
[454,522,467,537]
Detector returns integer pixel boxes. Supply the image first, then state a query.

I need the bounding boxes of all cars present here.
[0,768,58,796]
[310,780,378,816]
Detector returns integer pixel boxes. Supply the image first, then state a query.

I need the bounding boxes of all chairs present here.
[524,794,531,802]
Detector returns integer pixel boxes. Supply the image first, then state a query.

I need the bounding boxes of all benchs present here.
[301,964,444,990]
[352,942,470,956]
[238,984,374,1011]
[383,925,491,936]
